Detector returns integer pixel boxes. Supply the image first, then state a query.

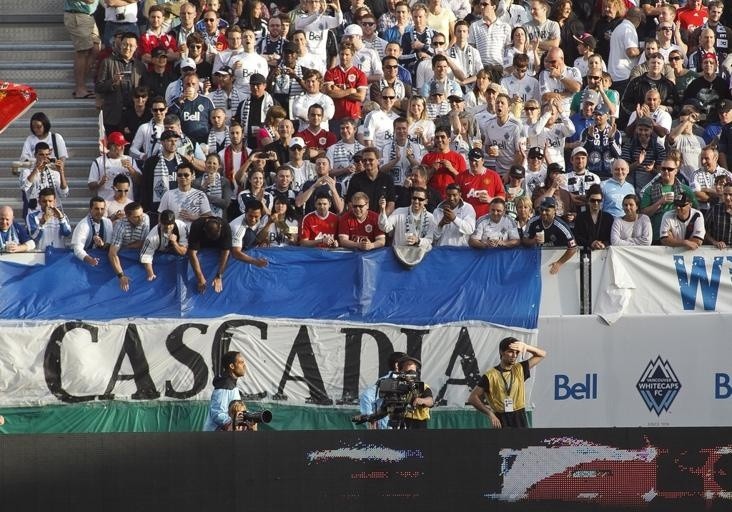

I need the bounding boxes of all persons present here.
[353,7,369,29]
[335,24,384,109]
[121,86,154,155]
[520,196,577,275]
[574,183,614,249]
[494,0,530,29]
[668,49,700,101]
[703,99,732,174]
[398,164,442,213]
[211,25,245,88]
[448,110,483,170]
[538,47,583,119]
[639,149,691,202]
[564,88,601,154]
[443,20,485,94]
[406,94,437,152]
[648,4,689,54]
[370,55,413,119]
[338,191,386,251]
[465,0,483,25]
[401,4,438,97]
[468,198,522,249]
[87,132,143,202]
[263,165,299,221]
[630,37,676,86]
[424,0,458,52]
[163,114,207,179]
[382,117,429,194]
[562,147,601,213]
[609,194,653,246]
[547,0,572,30]
[416,33,467,97]
[532,163,580,230]
[688,28,731,86]
[659,192,706,250]
[326,29,338,70]
[259,195,299,247]
[378,0,415,32]
[572,33,608,78]
[527,92,576,174]
[263,119,295,170]
[324,117,366,198]
[194,0,230,66]
[462,68,493,114]
[95,31,149,141]
[638,155,699,246]
[683,52,732,128]
[224,400,258,432]
[673,0,710,45]
[657,21,688,68]
[292,70,335,132]
[620,117,668,197]
[266,42,308,138]
[569,68,617,117]
[432,183,477,247]
[363,86,402,170]
[345,146,397,217]
[467,0,512,84]
[18,142,70,214]
[139,47,178,103]
[293,104,337,163]
[385,41,412,85]
[108,201,151,292]
[524,99,540,151]
[256,15,291,94]
[591,0,626,69]
[173,32,213,83]
[515,195,536,239]
[228,200,270,267]
[129,96,168,172]
[502,26,537,77]
[234,73,281,150]
[203,351,247,431]
[70,196,113,267]
[336,0,374,45]
[641,0,680,40]
[421,126,467,201]
[95,30,126,155]
[581,54,603,91]
[187,216,232,295]
[689,145,732,216]
[579,103,622,181]
[359,352,408,429]
[730,0,732,56]
[62,0,102,100]
[468,337,547,429]
[167,3,208,67]
[664,104,707,188]
[237,168,274,216]
[503,165,526,219]
[534,51,548,80]
[294,0,344,69]
[102,174,135,226]
[522,147,548,200]
[600,73,621,126]
[164,57,212,105]
[192,152,232,224]
[196,0,230,33]
[287,0,308,39]
[521,0,561,79]
[424,80,452,121]
[381,2,416,48]
[255,105,286,152]
[340,150,365,198]
[165,72,216,153]
[456,147,506,218]
[481,92,525,187]
[206,65,249,128]
[704,182,732,250]
[138,5,180,71]
[607,7,643,102]
[141,130,190,230]
[435,90,480,141]
[277,13,291,39]
[226,29,269,98]
[475,82,515,131]
[216,120,253,225]
[420,54,464,101]
[500,53,540,123]
[26,188,72,250]
[236,0,271,45]
[139,209,190,282]
[0,206,36,253]
[625,90,673,153]
[598,159,636,219]
[18,111,70,222]
[99,0,140,47]
[0,416,5,425]
[295,156,345,218]
[292,30,328,81]
[283,137,318,217]
[299,192,341,248]
[377,187,435,248]
[234,147,281,190]
[156,163,211,235]
[200,107,231,157]
[701,175,732,217]
[619,52,684,132]
[350,356,435,430]
[324,41,368,135]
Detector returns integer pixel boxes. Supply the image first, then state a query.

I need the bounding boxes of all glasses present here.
[515,66,527,72]
[590,198,602,203]
[433,42,446,45]
[545,60,556,65]
[351,203,367,208]
[113,186,130,192]
[413,197,426,202]
[658,26,673,31]
[382,96,395,99]
[152,106,166,112]
[524,107,539,110]
[661,167,675,172]
[589,76,603,80]
[176,173,191,177]
[479,2,492,6]
[669,56,681,62]
[384,65,398,69]
[204,18,217,22]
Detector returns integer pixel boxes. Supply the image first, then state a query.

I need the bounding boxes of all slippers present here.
[72,91,96,98]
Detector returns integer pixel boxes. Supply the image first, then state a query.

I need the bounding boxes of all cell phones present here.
[46,207,55,215]
[45,157,57,164]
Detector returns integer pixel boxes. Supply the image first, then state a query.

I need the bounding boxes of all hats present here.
[344,24,362,35]
[108,132,129,145]
[718,99,732,112]
[573,33,596,50]
[592,104,609,115]
[289,137,304,148]
[151,47,167,57]
[674,192,691,205]
[161,131,181,139]
[467,149,484,160]
[249,74,266,83]
[283,42,297,52]
[214,66,233,75]
[547,163,563,172]
[509,165,525,178]
[583,90,598,104]
[362,22,376,26]
[571,147,587,156]
[180,58,196,69]
[486,83,500,91]
[537,197,554,207]
[637,117,653,127]
[429,81,445,95]
[448,92,464,100]
[703,53,716,60]
[160,210,174,223]
[528,147,544,155]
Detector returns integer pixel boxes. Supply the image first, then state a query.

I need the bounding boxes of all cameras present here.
[233,410,273,426]
[258,152,269,158]
[279,69,291,75]
[114,12,125,23]
[326,0,332,3]
[352,370,424,430]
